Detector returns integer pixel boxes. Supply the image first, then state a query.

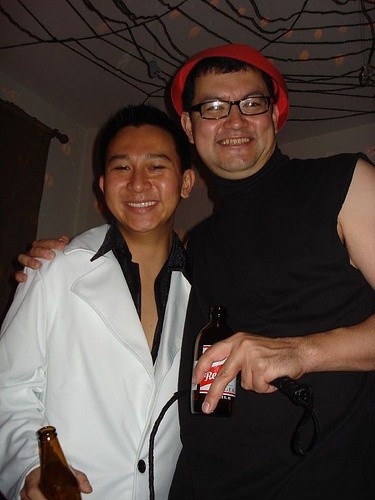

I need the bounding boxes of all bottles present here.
[191,306,237,418]
[36,426,82,500]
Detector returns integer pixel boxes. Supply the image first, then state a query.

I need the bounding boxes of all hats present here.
[171,44,289,130]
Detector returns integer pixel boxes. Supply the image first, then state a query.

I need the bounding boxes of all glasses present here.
[191,96,272,119]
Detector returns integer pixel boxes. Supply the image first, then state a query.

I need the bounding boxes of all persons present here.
[15,44,375,500]
[0,103,196,500]
